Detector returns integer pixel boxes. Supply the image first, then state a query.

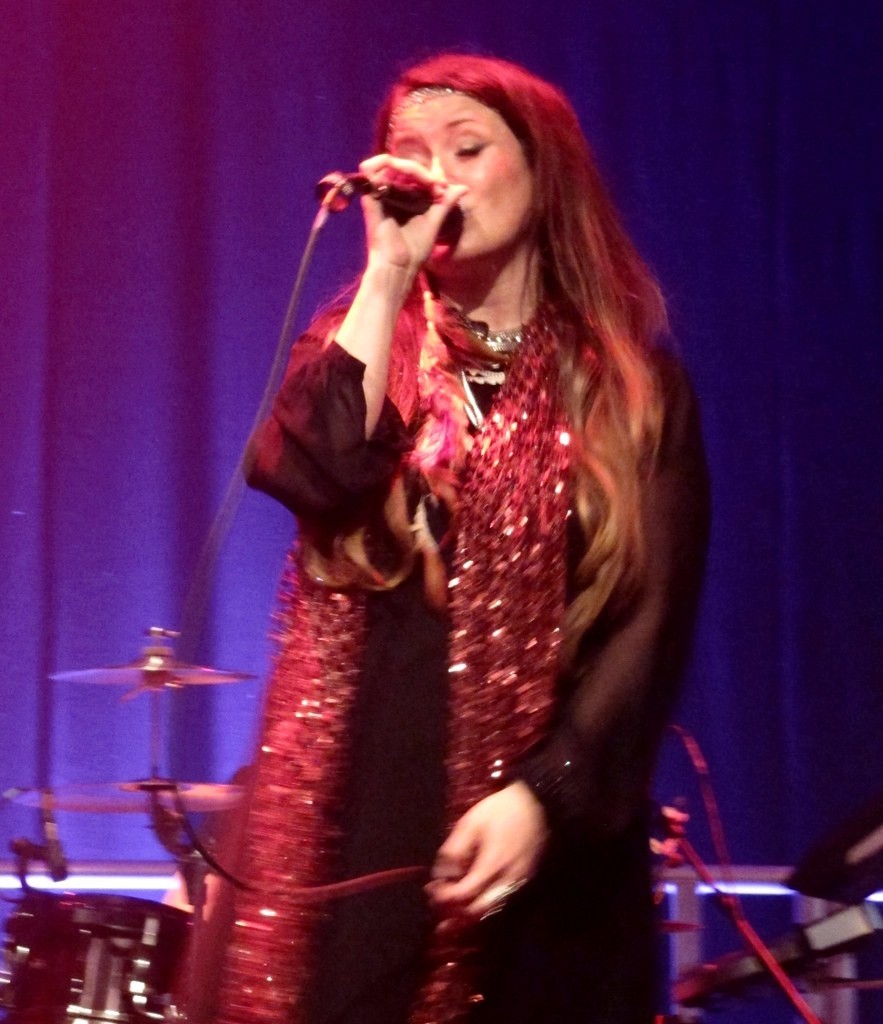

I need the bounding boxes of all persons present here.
[162,53,717,1023]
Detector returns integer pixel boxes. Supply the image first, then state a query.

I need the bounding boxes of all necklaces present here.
[454,319,533,373]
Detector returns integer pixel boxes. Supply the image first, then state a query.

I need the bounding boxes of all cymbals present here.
[45,655,257,686]
[3,776,251,813]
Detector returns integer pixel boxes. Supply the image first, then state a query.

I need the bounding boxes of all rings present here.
[481,877,528,922]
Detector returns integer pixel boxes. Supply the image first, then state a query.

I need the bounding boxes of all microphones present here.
[370,182,465,239]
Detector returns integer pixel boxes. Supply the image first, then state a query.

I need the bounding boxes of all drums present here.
[10,894,200,1020]
